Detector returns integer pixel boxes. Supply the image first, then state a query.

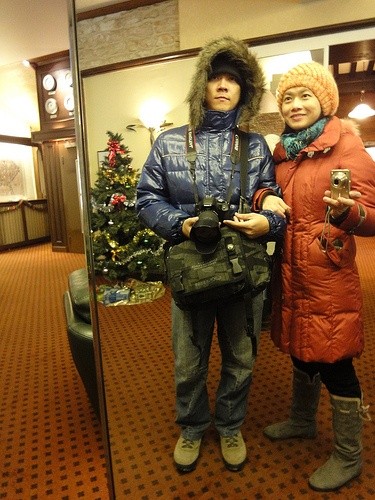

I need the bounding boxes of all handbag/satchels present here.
[163,203,271,312]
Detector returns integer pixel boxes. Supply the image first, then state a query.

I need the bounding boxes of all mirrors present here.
[68,0,375,500]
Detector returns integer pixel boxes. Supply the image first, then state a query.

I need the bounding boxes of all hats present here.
[275,61,339,122]
[206,57,245,90]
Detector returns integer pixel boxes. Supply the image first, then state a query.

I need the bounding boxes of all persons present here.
[133,37,375,491]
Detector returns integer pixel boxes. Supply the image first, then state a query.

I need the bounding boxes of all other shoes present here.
[174,432,201,473]
[219,429,247,471]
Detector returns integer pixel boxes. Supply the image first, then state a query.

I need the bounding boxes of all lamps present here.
[130,101,175,150]
[346,89,375,119]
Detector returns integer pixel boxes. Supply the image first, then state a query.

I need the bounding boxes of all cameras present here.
[330,169,349,199]
[189,197,225,255]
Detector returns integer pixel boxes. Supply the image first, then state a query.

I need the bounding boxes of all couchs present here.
[63,269,100,423]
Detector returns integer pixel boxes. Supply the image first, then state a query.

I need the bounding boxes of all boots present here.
[308,390,372,492]
[263,367,320,440]
